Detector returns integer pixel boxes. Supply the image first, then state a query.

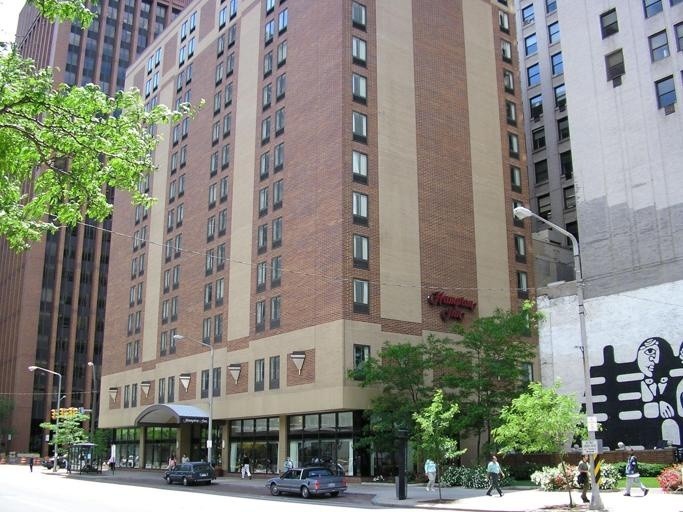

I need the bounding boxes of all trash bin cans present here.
[395,475,407,500]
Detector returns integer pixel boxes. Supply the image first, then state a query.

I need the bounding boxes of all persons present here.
[284,457,293,472]
[28,458,33,472]
[577,453,590,503]
[181,453,190,463]
[424,459,437,492]
[265,456,275,475]
[486,455,505,497]
[168,455,176,465]
[241,452,252,480]
[622,451,649,496]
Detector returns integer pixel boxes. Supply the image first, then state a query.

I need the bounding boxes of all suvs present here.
[208,459,238,472]
[161,462,215,485]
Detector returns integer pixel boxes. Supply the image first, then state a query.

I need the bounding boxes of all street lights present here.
[509,206,606,510]
[87,361,97,443]
[26,365,65,471]
[172,333,216,462]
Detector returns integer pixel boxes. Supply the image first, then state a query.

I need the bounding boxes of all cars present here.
[124,454,134,467]
[266,464,348,499]
[304,462,344,477]
[42,452,67,469]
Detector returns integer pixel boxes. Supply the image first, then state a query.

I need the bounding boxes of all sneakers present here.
[624,494,630,495]
[487,493,492,496]
[431,488,435,492]
[644,489,649,495]
[500,493,504,497]
[249,476,252,480]
[583,499,590,503]
[241,478,245,480]
[581,496,587,501]
[425,488,430,491]
